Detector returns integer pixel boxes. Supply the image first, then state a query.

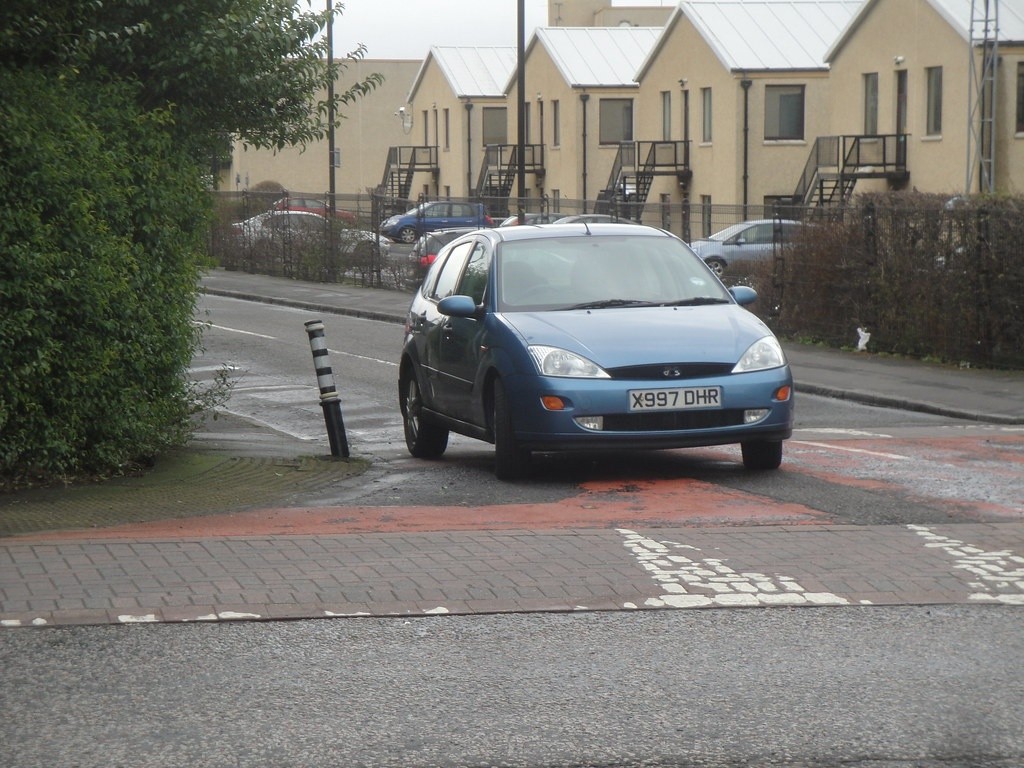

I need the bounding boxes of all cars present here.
[682,219,823,281]
[397,222,793,484]
[379,200,493,245]
[407,225,495,273]
[553,215,637,226]
[233,211,390,261]
[499,212,563,227]
[273,198,357,224]
